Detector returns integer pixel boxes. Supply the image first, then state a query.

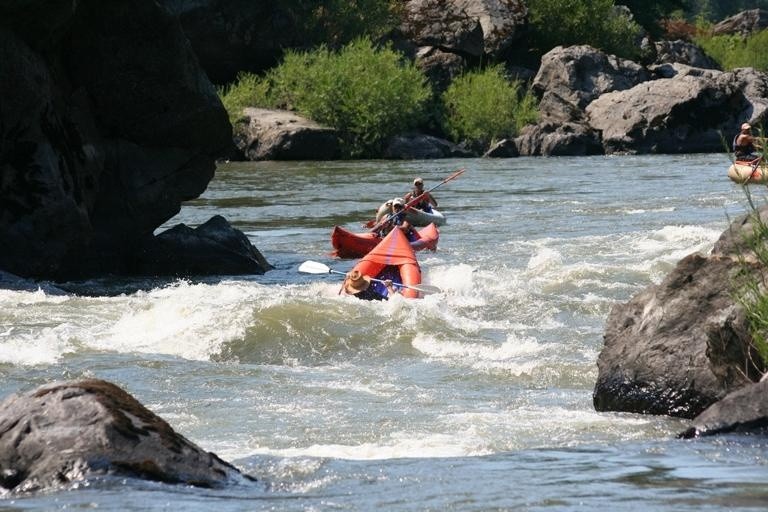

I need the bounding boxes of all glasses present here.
[415,183,422,185]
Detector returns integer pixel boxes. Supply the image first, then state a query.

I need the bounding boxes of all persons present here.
[360,196,420,244]
[402,176,437,214]
[729,121,767,164]
[342,270,395,301]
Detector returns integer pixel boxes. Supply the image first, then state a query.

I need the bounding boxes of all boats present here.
[729,161,767,182]
[332,222,440,253]
[339,225,421,298]
[377,197,445,226]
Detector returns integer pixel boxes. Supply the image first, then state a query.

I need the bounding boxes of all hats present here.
[345,271,371,295]
[414,177,423,185]
[393,198,405,207]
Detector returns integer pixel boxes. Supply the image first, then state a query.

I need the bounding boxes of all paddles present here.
[367,167,466,232]
[298,259,441,295]
